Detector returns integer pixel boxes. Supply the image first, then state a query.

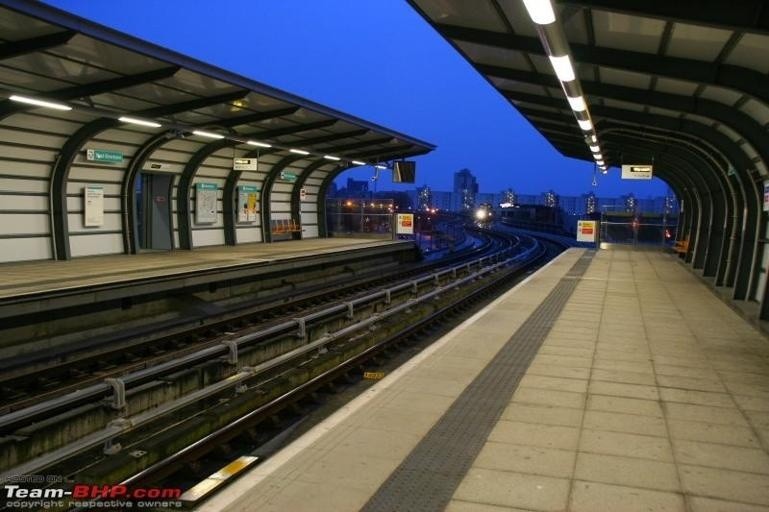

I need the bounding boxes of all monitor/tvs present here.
[392,160,416,183]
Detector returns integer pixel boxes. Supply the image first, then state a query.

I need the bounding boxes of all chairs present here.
[272,219,305,234]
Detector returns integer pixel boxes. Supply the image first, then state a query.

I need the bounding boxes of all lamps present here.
[522,0,608,174]
